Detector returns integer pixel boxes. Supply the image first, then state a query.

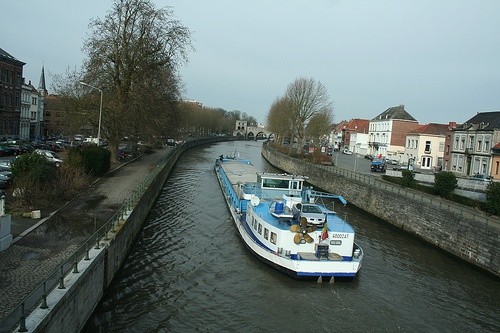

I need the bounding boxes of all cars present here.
[1,133,92,188]
[393,162,415,171]
[277,130,400,166]
[473,172,492,183]
[370,155,388,172]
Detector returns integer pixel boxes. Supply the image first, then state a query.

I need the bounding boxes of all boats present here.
[213,148,366,282]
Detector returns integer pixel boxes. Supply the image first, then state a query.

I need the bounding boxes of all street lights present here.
[78,81,103,147]
